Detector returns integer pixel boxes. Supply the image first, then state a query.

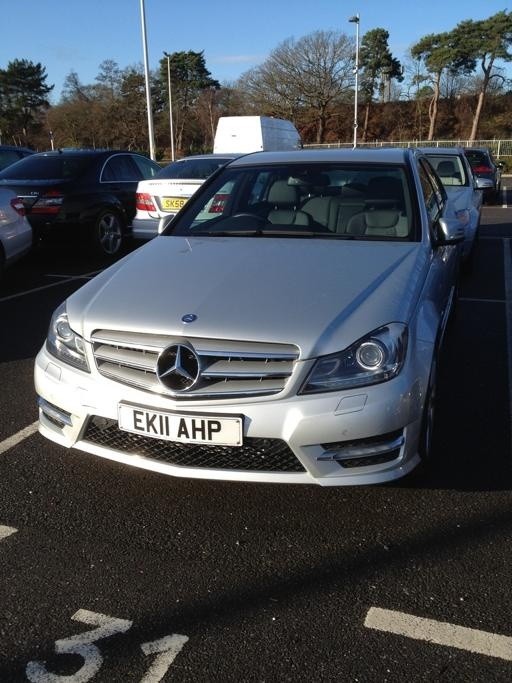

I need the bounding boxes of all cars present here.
[131,155,245,240]
[1,145,166,264]
[34,150,459,489]
[459,144,506,200]
[415,147,484,262]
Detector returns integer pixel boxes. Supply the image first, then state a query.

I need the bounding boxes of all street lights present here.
[162,50,184,163]
[348,14,361,146]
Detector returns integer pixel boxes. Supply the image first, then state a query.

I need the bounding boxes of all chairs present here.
[254,179,408,237]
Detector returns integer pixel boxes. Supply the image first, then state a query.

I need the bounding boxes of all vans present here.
[215,116,303,156]
